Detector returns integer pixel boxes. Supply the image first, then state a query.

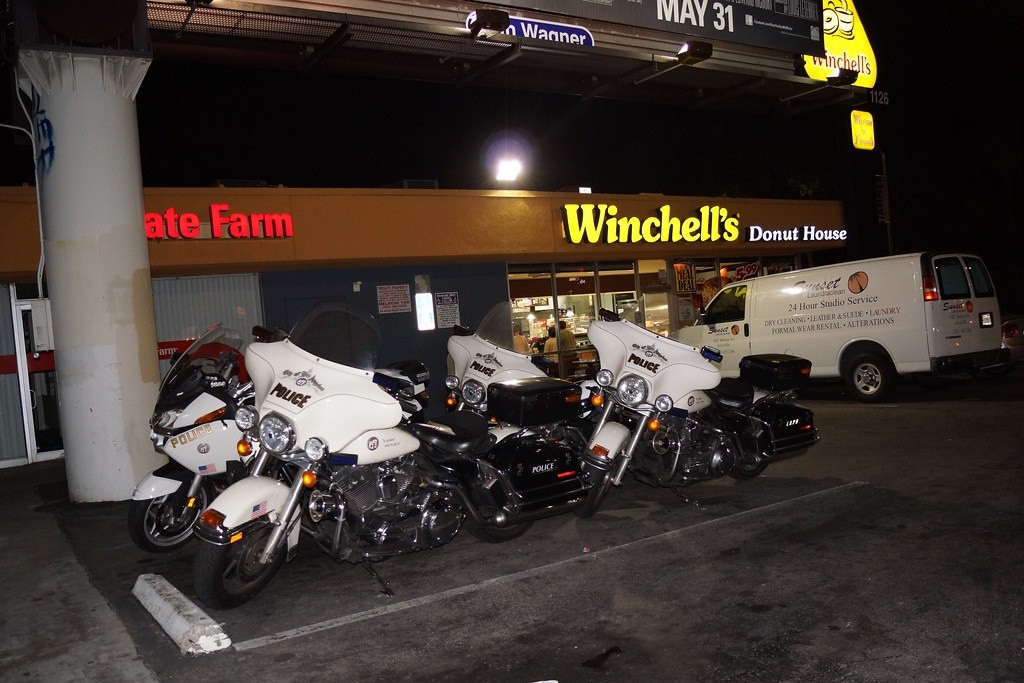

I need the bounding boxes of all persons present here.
[544,327,558,378]
[513,326,530,354]
[559,321,577,379]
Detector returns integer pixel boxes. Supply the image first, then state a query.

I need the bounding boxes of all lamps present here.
[827,67,859,87]
[466,9,510,39]
[676,40,713,65]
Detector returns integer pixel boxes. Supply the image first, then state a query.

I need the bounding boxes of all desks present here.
[573,361,601,367]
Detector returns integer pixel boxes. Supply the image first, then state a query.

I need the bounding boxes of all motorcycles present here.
[124,260,820,610]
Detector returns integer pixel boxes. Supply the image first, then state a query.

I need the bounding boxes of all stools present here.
[567,374,589,381]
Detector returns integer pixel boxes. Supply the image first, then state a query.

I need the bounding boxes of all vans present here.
[679,248,1011,402]
